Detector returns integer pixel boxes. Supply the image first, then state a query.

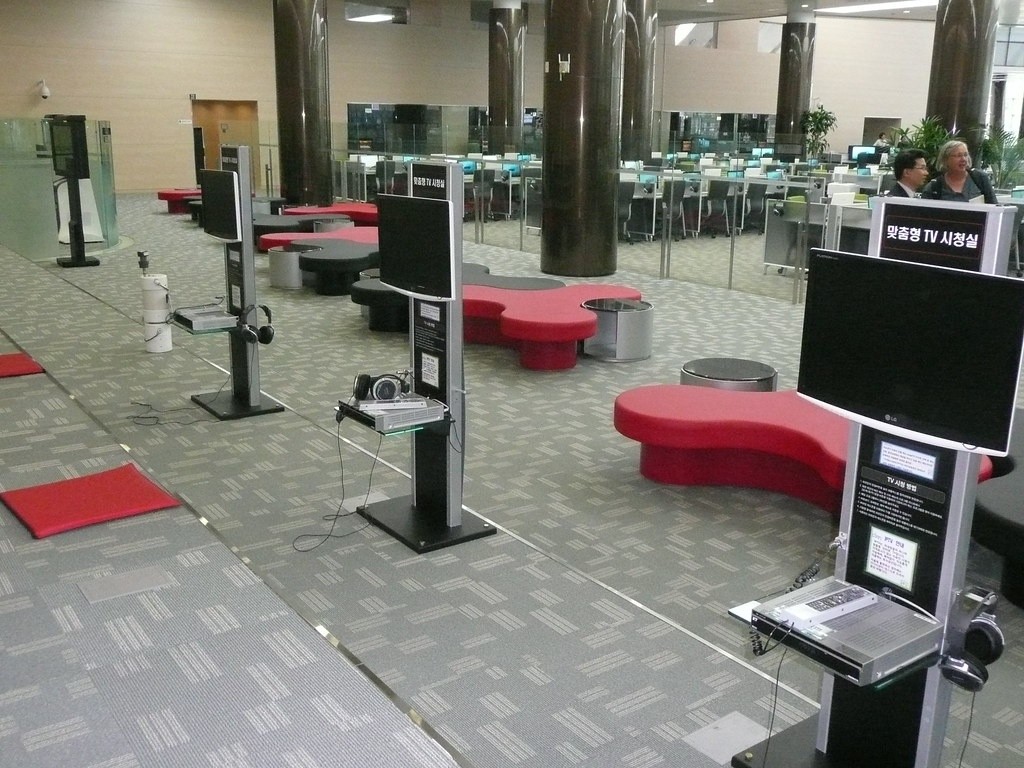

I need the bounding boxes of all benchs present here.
[352,263,641,370]
[158,188,380,296]
[613,385,1024,608]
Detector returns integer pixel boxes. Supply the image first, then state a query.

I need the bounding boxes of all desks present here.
[346,153,1024,224]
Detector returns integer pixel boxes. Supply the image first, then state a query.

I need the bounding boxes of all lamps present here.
[36,79,50,99]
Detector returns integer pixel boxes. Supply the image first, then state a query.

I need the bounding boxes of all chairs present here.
[375,160,396,194]
[736,183,767,236]
[999,203,1024,277]
[511,168,542,219]
[648,180,686,242]
[786,179,807,196]
[618,182,635,245]
[697,180,731,238]
[465,170,496,223]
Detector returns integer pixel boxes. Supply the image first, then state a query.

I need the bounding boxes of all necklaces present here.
[945,171,968,186]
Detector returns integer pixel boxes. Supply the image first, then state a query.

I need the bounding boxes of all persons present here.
[922,139,999,204]
[884,148,929,199]
[872,132,889,146]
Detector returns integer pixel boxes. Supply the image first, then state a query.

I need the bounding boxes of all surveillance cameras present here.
[40,86,50,99]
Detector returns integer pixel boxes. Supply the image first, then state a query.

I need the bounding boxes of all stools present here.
[281,203,318,216]
[269,246,320,289]
[314,220,355,234]
[360,269,379,317]
[680,359,779,393]
[583,298,653,364]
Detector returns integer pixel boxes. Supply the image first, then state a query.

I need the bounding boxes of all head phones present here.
[238,303,274,344]
[353,373,411,404]
[939,585,1005,693]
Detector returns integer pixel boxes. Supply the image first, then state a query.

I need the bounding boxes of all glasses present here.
[915,167,929,171]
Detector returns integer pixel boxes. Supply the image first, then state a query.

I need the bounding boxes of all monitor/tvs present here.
[52,127,75,172]
[797,248,1024,458]
[199,169,242,243]
[375,193,457,302]
[852,146,876,161]
